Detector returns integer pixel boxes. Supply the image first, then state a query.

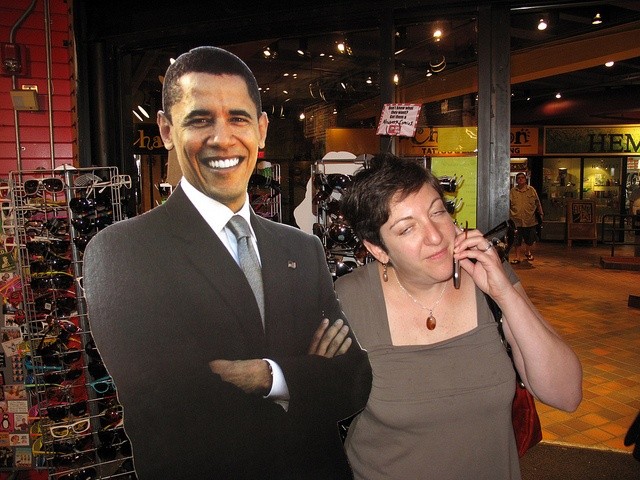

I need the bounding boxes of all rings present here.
[476,241,493,252]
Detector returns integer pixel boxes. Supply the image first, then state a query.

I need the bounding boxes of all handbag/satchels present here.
[511,386,542,459]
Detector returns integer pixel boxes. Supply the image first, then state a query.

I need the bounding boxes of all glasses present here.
[50,419,90,437]
[159,186,172,199]
[86,175,132,193]
[311,192,346,222]
[96,442,133,460]
[31,273,73,292]
[484,218,518,264]
[85,341,101,358]
[92,382,116,393]
[97,428,130,444]
[71,215,113,233]
[27,241,71,255]
[76,276,83,289]
[73,236,91,254]
[35,297,78,316]
[0,201,10,218]
[36,322,70,355]
[29,258,71,274]
[9,291,25,305]
[0,275,22,297]
[58,469,98,480]
[14,310,43,324]
[0,236,15,250]
[312,222,335,250]
[442,201,453,216]
[68,197,112,213]
[44,368,83,383]
[23,376,64,392]
[24,178,64,195]
[105,407,124,426]
[335,259,354,277]
[439,179,456,192]
[44,347,81,366]
[32,441,60,455]
[329,224,368,258]
[30,424,49,440]
[19,325,46,341]
[24,358,61,370]
[48,399,87,421]
[51,453,93,470]
[1,220,13,233]
[28,400,70,424]
[89,360,105,379]
[35,456,69,471]
[265,213,279,222]
[314,173,353,190]
[18,343,34,356]
[55,438,92,452]
[0,182,10,197]
[25,217,69,236]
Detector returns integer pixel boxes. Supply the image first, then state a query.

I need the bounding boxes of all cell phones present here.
[453,251,461,288]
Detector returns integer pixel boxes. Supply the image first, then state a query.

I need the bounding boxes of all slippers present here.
[525,253,534,261]
[511,259,521,264]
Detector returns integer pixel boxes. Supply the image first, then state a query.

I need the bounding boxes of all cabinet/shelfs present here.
[246,162,282,223]
[311,155,431,281]
[0,167,138,479]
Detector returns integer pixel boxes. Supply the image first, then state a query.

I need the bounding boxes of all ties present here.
[226,214,265,331]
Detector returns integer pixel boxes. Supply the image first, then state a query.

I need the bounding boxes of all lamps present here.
[394,35,408,56]
[337,36,353,55]
[263,41,278,57]
[296,40,307,56]
[425,45,449,72]
[393,63,406,86]
[366,76,373,84]
[591,12,603,25]
[332,108,338,115]
[553,90,567,101]
[133,101,155,121]
[536,18,548,32]
[340,82,349,90]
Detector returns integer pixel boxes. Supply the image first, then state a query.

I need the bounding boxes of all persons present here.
[510,172,545,265]
[306,151,585,479]
[82,45,374,478]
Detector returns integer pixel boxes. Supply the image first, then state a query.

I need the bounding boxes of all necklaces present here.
[390,267,451,331]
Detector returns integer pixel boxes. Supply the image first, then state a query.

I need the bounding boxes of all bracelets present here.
[540,214,544,216]
